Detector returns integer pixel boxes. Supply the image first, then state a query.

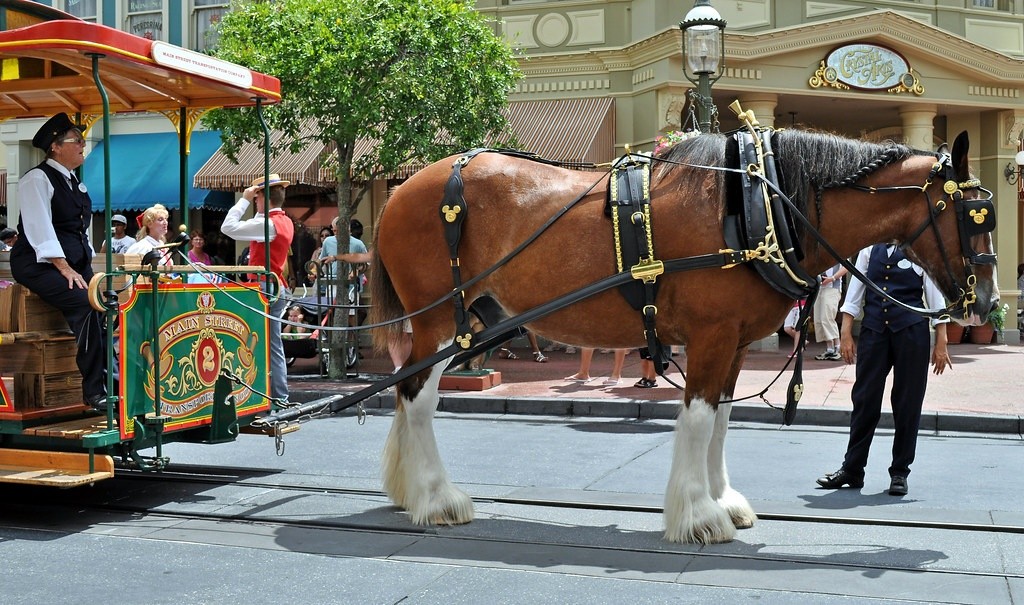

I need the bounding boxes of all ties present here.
[66,172,83,207]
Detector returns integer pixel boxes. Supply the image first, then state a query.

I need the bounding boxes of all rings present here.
[74,279,78,280]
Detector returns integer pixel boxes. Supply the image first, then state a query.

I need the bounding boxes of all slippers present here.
[564,373,590,382]
[598,379,619,386]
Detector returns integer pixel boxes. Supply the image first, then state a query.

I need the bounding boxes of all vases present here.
[971,322,994,343]
[947,322,964,344]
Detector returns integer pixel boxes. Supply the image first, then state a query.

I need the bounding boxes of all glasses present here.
[56,138,86,144]
[321,234,329,237]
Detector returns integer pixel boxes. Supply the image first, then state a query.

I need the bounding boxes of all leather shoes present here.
[83,387,116,410]
[888,474,909,496]
[815,467,865,489]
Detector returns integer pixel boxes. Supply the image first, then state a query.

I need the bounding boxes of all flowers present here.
[988,303,1010,339]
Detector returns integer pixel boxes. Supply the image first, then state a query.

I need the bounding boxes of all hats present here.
[137,211,151,233]
[111,215,127,224]
[251,174,290,193]
[32,113,87,154]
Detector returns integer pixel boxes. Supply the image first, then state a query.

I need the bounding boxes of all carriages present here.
[0,0,1001,550]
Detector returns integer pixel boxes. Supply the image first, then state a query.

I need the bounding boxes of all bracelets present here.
[831,275,836,282]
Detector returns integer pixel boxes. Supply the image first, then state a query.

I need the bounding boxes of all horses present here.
[364,126,999,545]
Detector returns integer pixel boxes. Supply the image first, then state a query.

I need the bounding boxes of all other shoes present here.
[624,349,631,355]
[600,348,610,354]
[542,344,560,352]
[564,346,577,354]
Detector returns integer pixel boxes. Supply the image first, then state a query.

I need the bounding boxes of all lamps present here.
[1004,151,1024,185]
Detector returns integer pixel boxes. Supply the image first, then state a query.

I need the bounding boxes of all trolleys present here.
[277,282,359,378]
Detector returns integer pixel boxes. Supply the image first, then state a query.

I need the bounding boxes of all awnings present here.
[73,97,617,208]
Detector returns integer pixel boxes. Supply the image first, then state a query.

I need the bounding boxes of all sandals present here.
[532,351,549,363]
[634,376,658,388]
[786,351,798,358]
[498,347,519,360]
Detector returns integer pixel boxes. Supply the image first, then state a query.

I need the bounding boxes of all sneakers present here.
[814,347,841,360]
[345,347,356,367]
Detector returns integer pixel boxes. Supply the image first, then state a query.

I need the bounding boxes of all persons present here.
[285,307,309,334]
[221,174,294,406]
[786,257,849,361]
[309,218,366,322]
[0,228,18,251]
[566,345,659,387]
[100,204,211,269]
[499,331,548,363]
[320,249,414,376]
[816,237,954,496]
[10,111,124,409]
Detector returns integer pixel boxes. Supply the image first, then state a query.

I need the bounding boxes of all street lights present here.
[674,0,729,134]
